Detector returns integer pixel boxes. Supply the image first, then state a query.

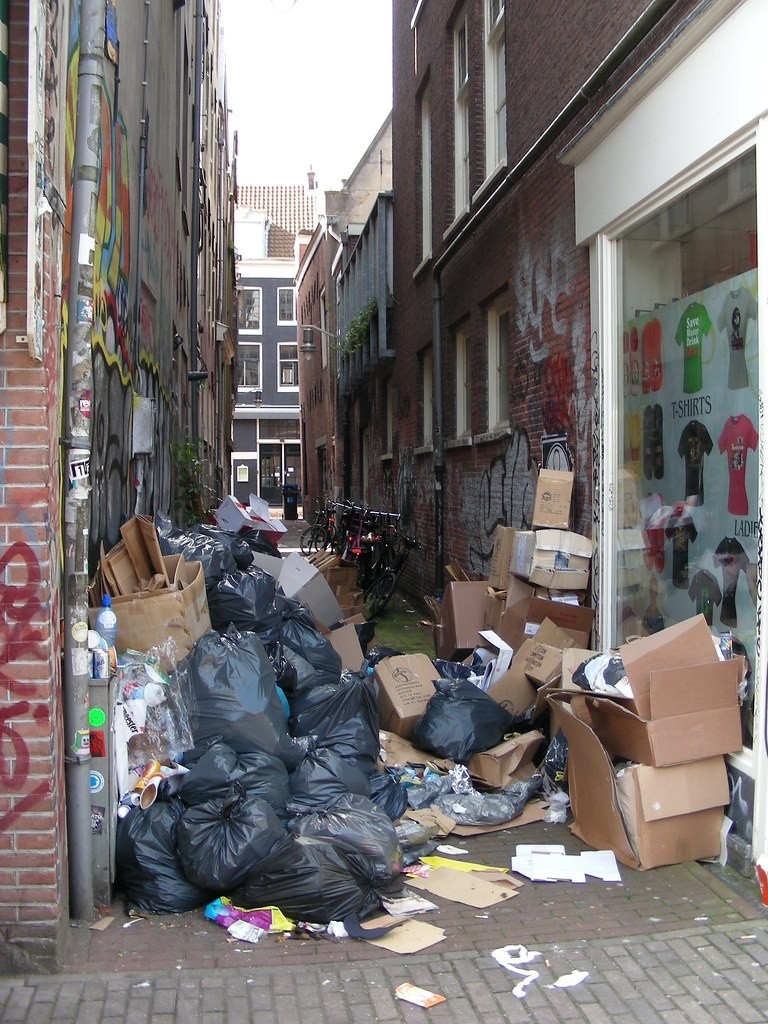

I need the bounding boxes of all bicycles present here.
[300,495,421,620]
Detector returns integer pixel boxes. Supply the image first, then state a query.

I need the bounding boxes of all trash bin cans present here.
[281,490,298,520]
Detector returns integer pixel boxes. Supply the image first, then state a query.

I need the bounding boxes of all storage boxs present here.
[89,460,746,870]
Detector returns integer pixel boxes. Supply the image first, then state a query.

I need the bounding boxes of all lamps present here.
[173,335,183,352]
[187,352,209,382]
[297,324,339,361]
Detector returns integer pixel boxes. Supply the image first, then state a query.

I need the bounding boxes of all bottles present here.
[95,595,116,648]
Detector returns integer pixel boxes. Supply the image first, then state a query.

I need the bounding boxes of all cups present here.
[88,630,108,650]
